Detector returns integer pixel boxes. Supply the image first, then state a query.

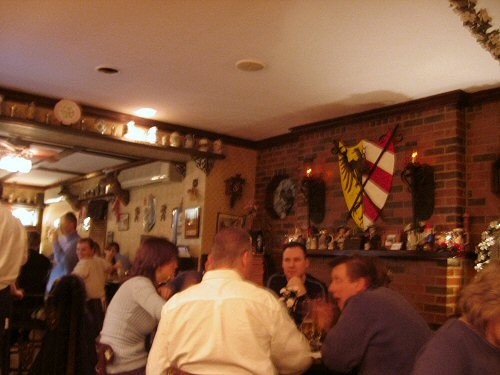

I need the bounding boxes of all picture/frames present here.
[184,207,200,238]
[117,213,129,231]
[215,212,246,232]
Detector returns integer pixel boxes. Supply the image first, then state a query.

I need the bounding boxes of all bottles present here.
[256,235,263,254]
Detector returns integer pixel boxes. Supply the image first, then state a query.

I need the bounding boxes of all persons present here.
[268,240,328,330]
[314,252,433,375]
[413,260,500,375]
[9,210,205,323]
[0,180,29,375]
[93,235,178,375]
[145,225,313,375]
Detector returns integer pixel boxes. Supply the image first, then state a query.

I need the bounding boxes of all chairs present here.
[94,336,115,375]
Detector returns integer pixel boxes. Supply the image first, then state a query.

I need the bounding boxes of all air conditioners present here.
[118,161,184,190]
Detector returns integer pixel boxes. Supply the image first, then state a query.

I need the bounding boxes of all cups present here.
[302,321,322,351]
